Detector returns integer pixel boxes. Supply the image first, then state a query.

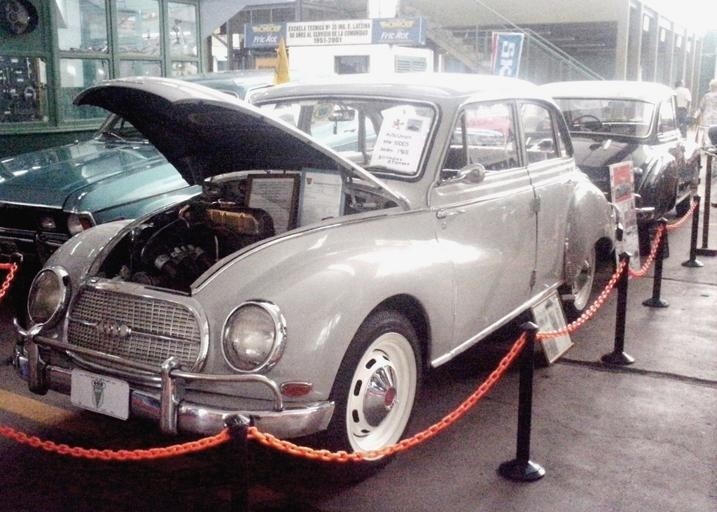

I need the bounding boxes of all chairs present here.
[445,148,554,168]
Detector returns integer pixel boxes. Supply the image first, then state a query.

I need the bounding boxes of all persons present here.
[674,80,692,141]
[695,79,717,145]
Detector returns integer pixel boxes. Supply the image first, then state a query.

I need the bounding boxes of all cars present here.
[540,80,700,257]
